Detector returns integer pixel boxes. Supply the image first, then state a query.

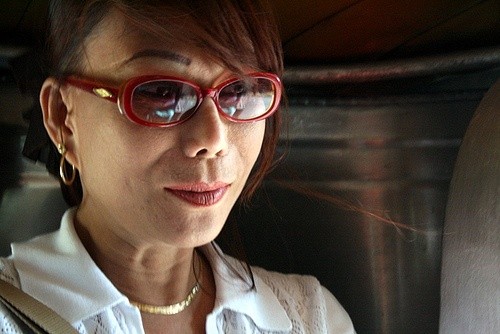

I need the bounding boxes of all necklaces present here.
[109,249,203,316]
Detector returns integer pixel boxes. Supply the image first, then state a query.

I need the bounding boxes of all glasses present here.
[61,71,285,129]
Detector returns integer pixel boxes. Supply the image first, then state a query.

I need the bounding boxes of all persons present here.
[0,1,357,334]
